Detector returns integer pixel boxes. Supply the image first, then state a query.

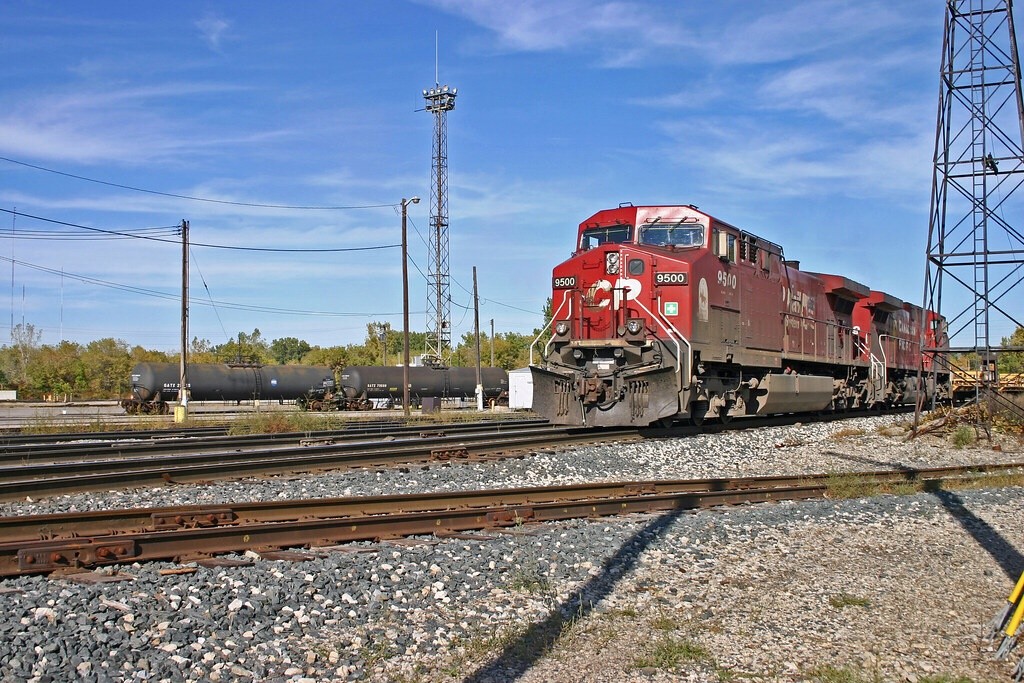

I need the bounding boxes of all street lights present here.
[401,196,420,415]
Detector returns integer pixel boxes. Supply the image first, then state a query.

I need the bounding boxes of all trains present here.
[529,201,952,427]
[118,332,510,414]
